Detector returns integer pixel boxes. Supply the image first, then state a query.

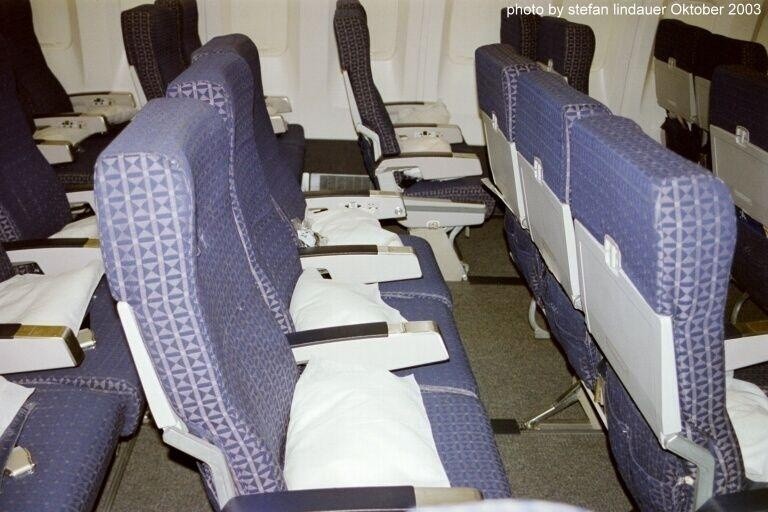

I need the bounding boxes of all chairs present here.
[1,1,766,511]
